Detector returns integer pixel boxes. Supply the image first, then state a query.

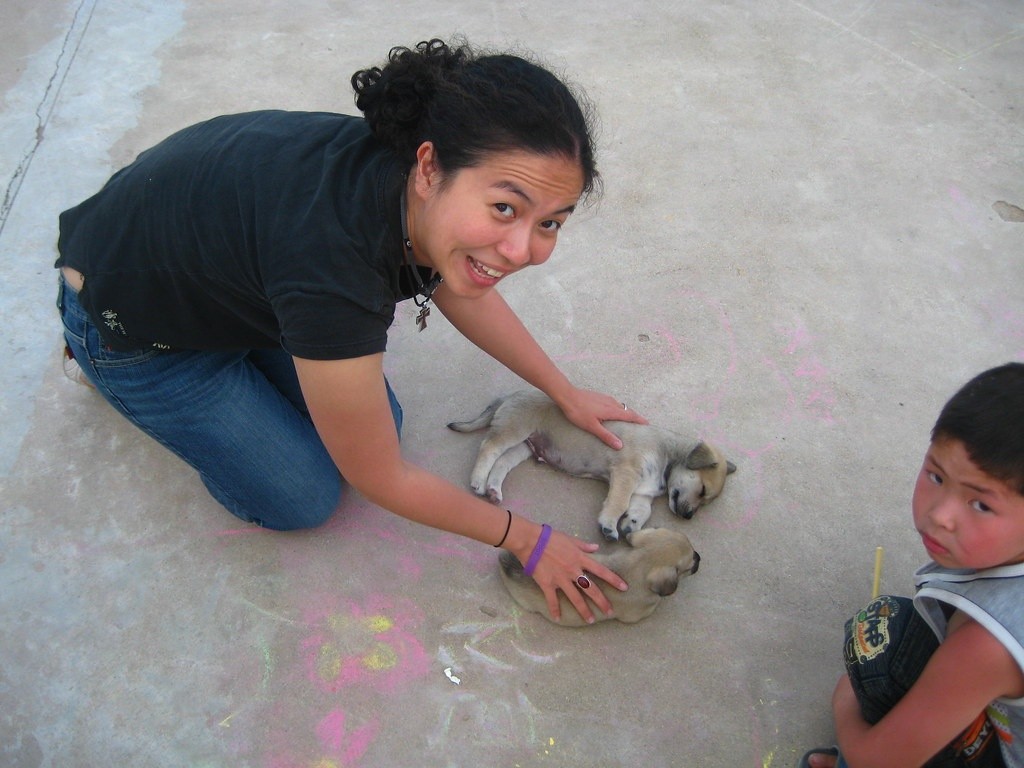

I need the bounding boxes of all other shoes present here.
[64,352,96,388]
[799,745,848,768]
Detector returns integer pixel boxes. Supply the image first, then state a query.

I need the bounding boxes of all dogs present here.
[447,389,737,540]
[497,526,702,627]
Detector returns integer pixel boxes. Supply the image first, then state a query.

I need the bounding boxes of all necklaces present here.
[396,186,444,333]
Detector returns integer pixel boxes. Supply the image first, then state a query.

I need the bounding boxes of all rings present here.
[576,574,591,590]
[622,402,628,410]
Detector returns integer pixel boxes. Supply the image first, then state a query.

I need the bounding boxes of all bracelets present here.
[524,523,552,577]
[493,509,512,547]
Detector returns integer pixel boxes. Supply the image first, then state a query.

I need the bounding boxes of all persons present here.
[50,38,652,626]
[799,361,1024,768]
